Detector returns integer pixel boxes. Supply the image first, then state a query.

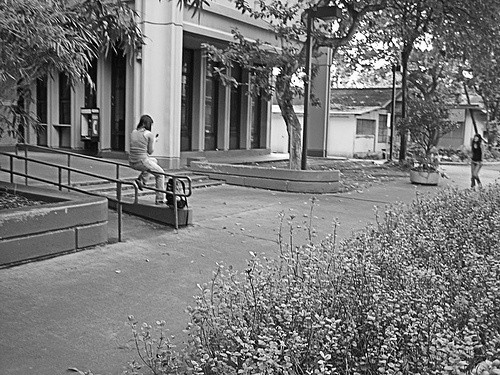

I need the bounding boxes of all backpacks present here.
[165,178,187,207]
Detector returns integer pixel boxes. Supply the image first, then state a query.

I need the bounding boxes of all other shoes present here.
[478,185,482,188]
[470,187,475,190]
[136,178,143,191]
[155,200,165,204]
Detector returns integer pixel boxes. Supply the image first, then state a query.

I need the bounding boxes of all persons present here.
[470,135,483,187]
[129,115,166,204]
